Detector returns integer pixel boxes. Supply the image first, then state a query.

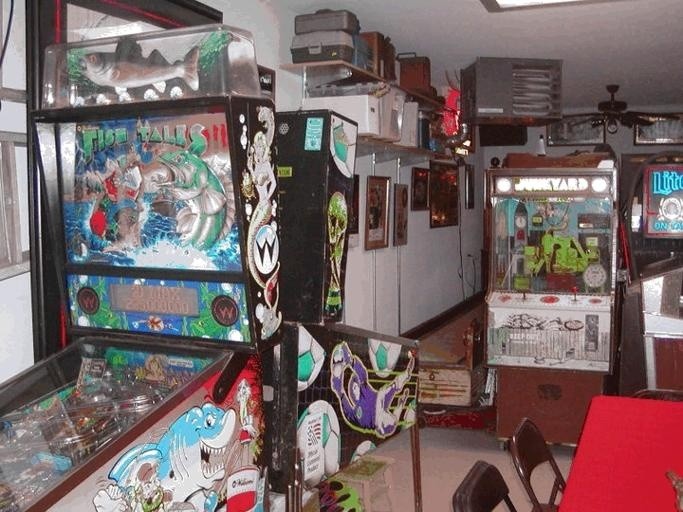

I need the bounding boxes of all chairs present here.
[450,415,567,511]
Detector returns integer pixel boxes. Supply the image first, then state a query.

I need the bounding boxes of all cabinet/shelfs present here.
[281,59,450,166]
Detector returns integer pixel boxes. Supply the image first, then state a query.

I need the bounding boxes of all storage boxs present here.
[289,9,431,96]
[302,84,431,150]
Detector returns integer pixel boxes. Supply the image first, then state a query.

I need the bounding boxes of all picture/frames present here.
[363,161,459,251]
[543,112,683,146]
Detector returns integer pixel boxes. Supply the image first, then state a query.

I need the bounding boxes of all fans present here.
[563,85,681,130]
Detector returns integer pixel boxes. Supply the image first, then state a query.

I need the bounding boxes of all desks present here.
[559,396,683,512]
